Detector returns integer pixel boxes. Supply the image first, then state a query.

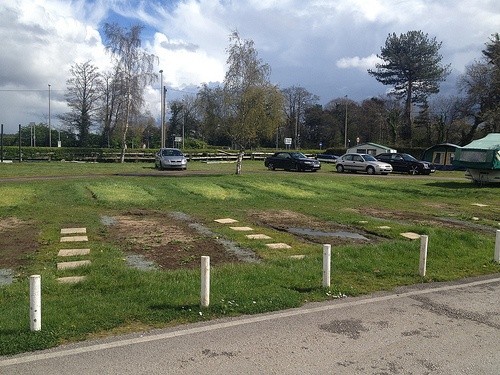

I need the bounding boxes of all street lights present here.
[182,99,185,149]
[344,94,347,149]
[48,83,52,147]
[159,69,164,148]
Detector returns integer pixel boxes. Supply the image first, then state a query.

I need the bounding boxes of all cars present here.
[373,152,437,175]
[264,152,321,172]
[154,147,190,171]
[335,153,393,176]
